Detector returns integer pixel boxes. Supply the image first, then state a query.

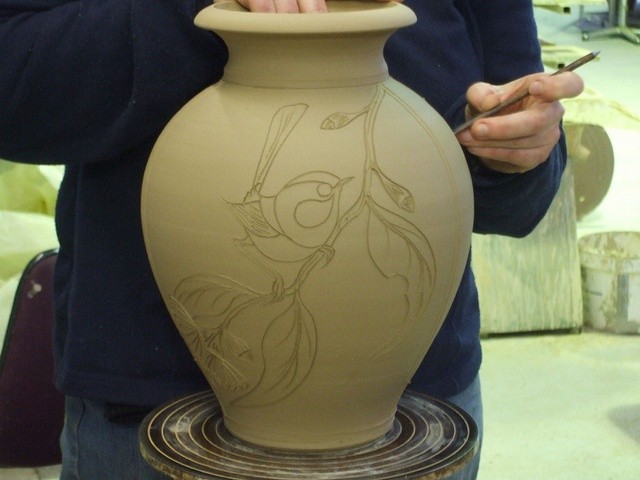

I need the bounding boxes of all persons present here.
[0,0,584,480]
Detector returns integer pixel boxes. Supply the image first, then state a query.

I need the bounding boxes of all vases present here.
[139,0,476,452]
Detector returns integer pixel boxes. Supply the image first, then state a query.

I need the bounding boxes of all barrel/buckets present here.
[579,231,640,335]
